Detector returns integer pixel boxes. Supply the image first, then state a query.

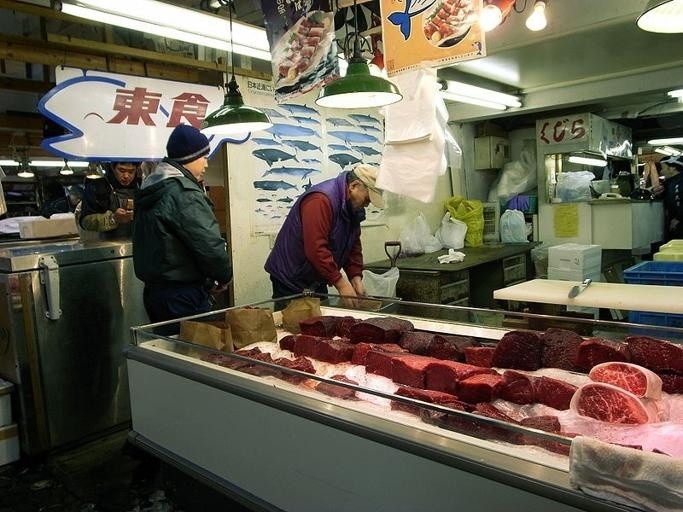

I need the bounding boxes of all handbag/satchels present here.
[630,189,654,200]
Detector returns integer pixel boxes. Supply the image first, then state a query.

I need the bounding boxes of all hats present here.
[353,164,384,209]
[166,123,210,164]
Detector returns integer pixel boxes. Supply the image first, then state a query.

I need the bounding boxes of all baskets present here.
[623,260,683,339]
[501,308,529,329]
[544,311,595,336]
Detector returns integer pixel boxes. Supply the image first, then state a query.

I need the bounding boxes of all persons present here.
[41,185,84,220]
[76,162,145,241]
[264,164,386,318]
[651,154,683,242]
[131,124,234,339]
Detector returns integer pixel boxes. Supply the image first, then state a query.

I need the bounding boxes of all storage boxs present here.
[546,242,601,315]
[622,260,683,338]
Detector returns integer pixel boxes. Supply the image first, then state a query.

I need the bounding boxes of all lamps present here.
[634,0,683,35]
[438,68,528,111]
[314,0,404,109]
[525,0,549,32]
[59,157,74,175]
[17,148,35,178]
[200,0,274,136]
[0,154,89,169]
[568,152,608,167]
[51,1,273,62]
[478,0,526,32]
[86,161,103,179]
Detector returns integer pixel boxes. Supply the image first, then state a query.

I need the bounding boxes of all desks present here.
[363,240,543,319]
[492,277,682,345]
